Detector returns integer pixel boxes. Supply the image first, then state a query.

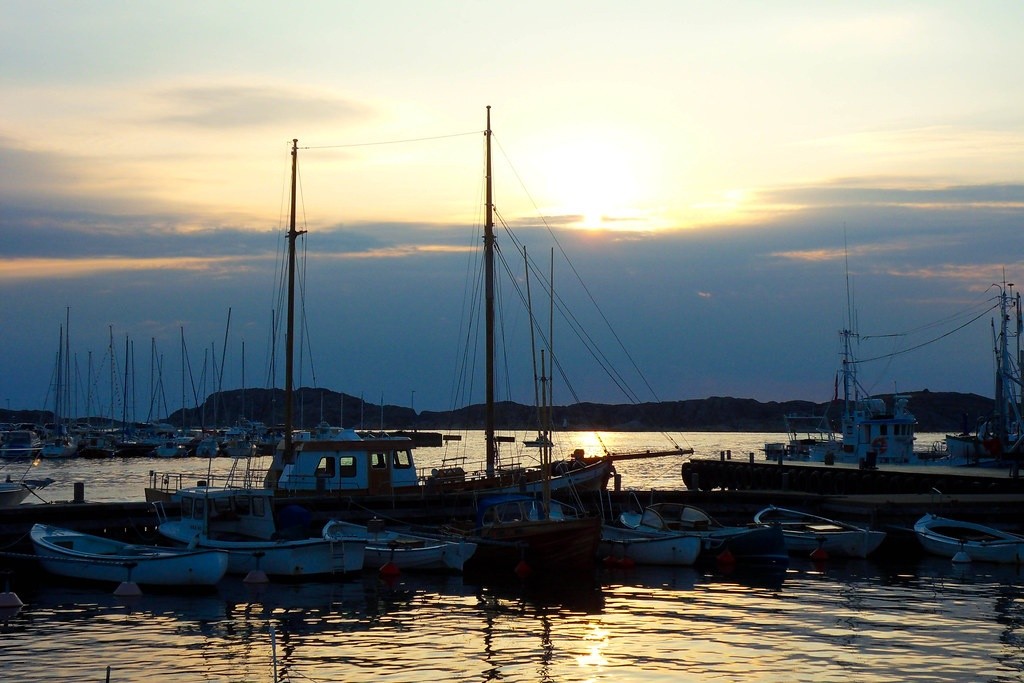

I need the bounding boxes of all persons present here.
[977,419,994,442]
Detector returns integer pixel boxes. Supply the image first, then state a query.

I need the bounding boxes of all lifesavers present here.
[872,437,887,453]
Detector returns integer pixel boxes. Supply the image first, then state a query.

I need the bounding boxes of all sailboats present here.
[0,103,622,550]
[0,305,390,463]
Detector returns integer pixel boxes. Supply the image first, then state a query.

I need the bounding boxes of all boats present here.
[29,522,228,587]
[762,442,787,455]
[752,503,886,563]
[619,501,789,569]
[533,495,701,567]
[157,486,367,576]
[790,279,1024,470]
[443,496,605,564]
[321,517,478,575]
[524,435,553,447]
[912,511,1024,564]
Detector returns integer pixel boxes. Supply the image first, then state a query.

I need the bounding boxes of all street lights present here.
[411,391,416,432]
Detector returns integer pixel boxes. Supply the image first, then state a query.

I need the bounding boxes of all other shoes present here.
[972,459,980,463]
